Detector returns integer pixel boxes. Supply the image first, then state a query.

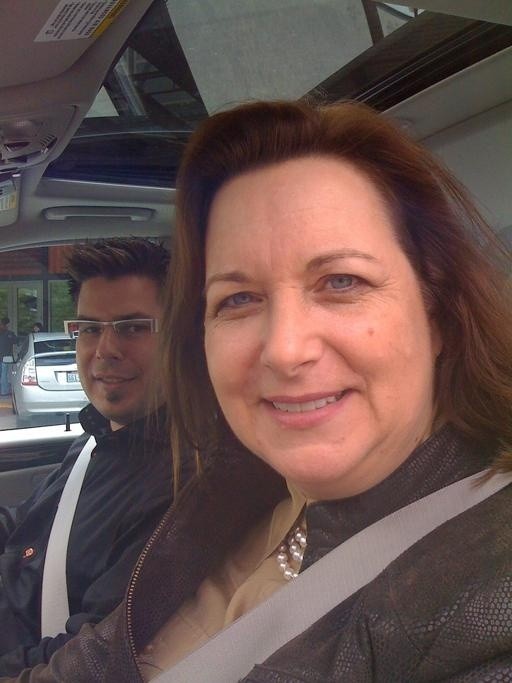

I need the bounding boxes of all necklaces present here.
[277,526,308,582]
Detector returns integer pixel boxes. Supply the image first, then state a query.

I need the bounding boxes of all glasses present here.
[64,317,164,341]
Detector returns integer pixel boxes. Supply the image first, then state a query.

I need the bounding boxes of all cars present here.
[2,331,89,425]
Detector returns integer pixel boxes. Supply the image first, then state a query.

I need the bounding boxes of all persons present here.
[0,318,18,396]
[0,235,204,676]
[32,323,42,332]
[2,98,512,683]
